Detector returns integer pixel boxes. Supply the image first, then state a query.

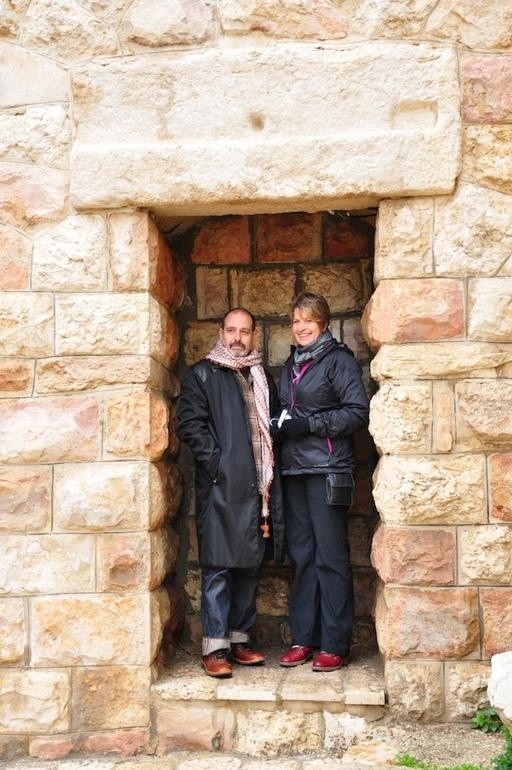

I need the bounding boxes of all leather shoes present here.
[279,644,313,667]
[201,649,232,677]
[228,642,265,666]
[312,648,349,671]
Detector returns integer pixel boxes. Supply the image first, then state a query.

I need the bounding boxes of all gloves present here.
[270,425,281,447]
[279,417,310,438]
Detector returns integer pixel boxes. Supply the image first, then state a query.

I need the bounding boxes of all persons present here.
[270,292,369,672]
[176,308,286,676]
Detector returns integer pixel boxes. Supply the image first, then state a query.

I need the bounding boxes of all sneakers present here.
[325,472,355,506]
